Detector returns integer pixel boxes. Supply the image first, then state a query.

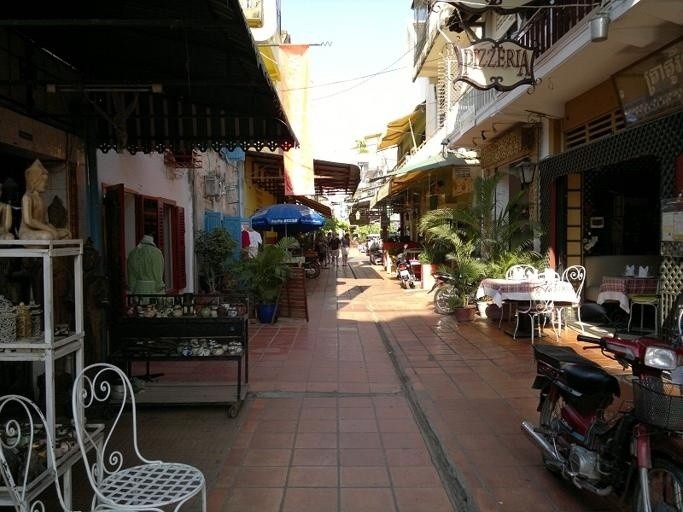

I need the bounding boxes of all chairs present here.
[626,275,661,335]
[541,264,586,333]
[70,363,208,512]
[0,393,81,511]
[498,264,539,331]
[512,270,561,344]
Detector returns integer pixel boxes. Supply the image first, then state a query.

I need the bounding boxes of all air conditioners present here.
[204,174,227,196]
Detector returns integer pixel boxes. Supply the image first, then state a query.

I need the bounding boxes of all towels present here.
[638,265,651,277]
[624,264,635,276]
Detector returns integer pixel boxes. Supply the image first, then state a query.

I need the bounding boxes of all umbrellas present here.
[248,200,325,253]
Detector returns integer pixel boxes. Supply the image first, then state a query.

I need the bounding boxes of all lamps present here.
[514,151,538,186]
[587,10,611,42]
[45,82,165,94]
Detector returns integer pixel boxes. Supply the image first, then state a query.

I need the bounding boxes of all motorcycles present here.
[426,271,485,315]
[388,243,415,289]
[302,256,320,279]
[520,303,683,512]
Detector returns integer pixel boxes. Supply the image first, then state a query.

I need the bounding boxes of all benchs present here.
[583,252,664,307]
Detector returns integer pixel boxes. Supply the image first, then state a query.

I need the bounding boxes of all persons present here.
[313,230,340,268]
[17,158,71,241]
[340,234,350,266]
[124,234,167,298]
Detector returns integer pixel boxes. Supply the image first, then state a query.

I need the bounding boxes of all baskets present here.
[632,378,683,431]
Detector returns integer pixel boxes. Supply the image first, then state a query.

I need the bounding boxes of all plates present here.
[622,273,655,280]
[508,277,558,283]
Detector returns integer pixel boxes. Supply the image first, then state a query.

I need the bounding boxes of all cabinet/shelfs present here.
[0,240,106,511]
[94,291,252,421]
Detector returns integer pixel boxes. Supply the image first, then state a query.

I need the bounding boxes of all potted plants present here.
[436,233,485,322]
[230,242,295,323]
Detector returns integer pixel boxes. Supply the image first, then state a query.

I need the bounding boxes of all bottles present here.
[176,338,241,358]
[0,420,84,488]
[0,293,42,345]
[128,304,183,318]
[200,303,245,319]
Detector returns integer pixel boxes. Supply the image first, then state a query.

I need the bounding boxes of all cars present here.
[357,234,382,265]
